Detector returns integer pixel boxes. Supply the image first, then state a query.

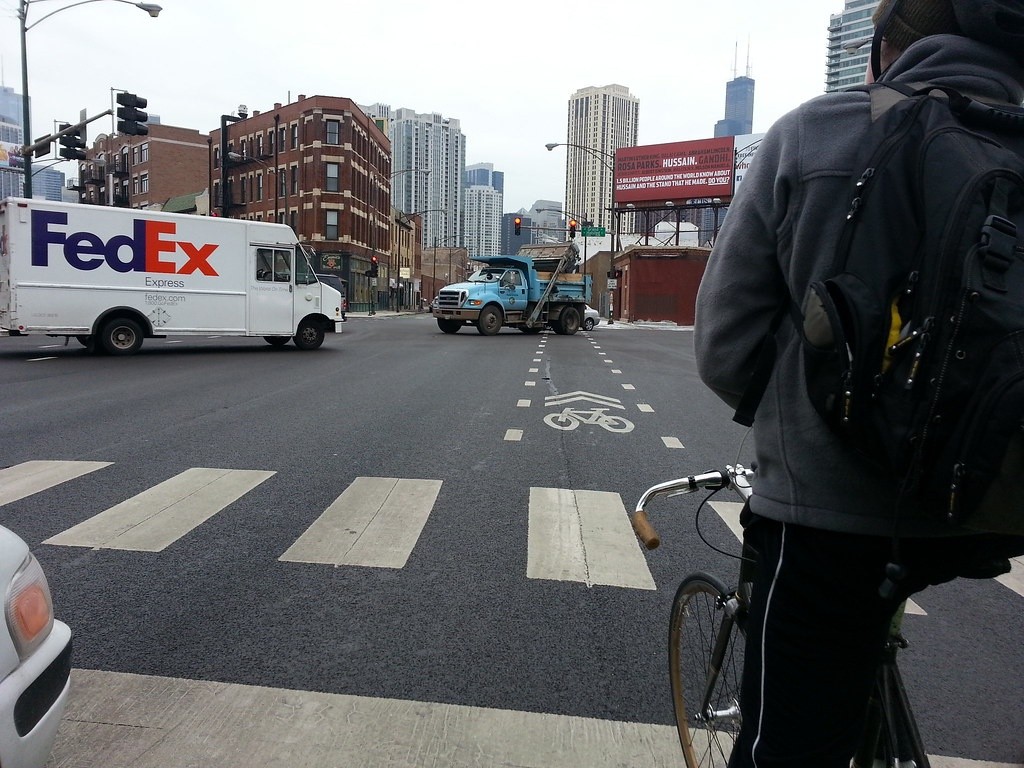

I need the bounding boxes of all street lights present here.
[396,209,449,313]
[17,0,162,199]
[536,208,588,333]
[546,142,616,324]
[431,234,471,299]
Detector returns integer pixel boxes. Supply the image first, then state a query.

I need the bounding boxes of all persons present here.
[694,0,1024,768]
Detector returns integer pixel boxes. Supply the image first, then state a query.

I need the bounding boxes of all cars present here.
[583,304,600,330]
[1,520,77,768]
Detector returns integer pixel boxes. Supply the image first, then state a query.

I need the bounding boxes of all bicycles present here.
[631,460,1015,768]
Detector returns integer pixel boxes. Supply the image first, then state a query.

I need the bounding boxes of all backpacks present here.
[769,72,1024,538]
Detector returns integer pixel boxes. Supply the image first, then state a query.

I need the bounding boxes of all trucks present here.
[0,194,348,358]
[430,253,594,337]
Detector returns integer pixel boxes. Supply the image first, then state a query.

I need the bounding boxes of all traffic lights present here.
[57,124,88,161]
[116,91,149,136]
[514,218,522,237]
[371,256,378,278]
[569,220,577,239]
[366,271,371,277]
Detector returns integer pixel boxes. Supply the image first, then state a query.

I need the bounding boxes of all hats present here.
[871,0,955,53]
